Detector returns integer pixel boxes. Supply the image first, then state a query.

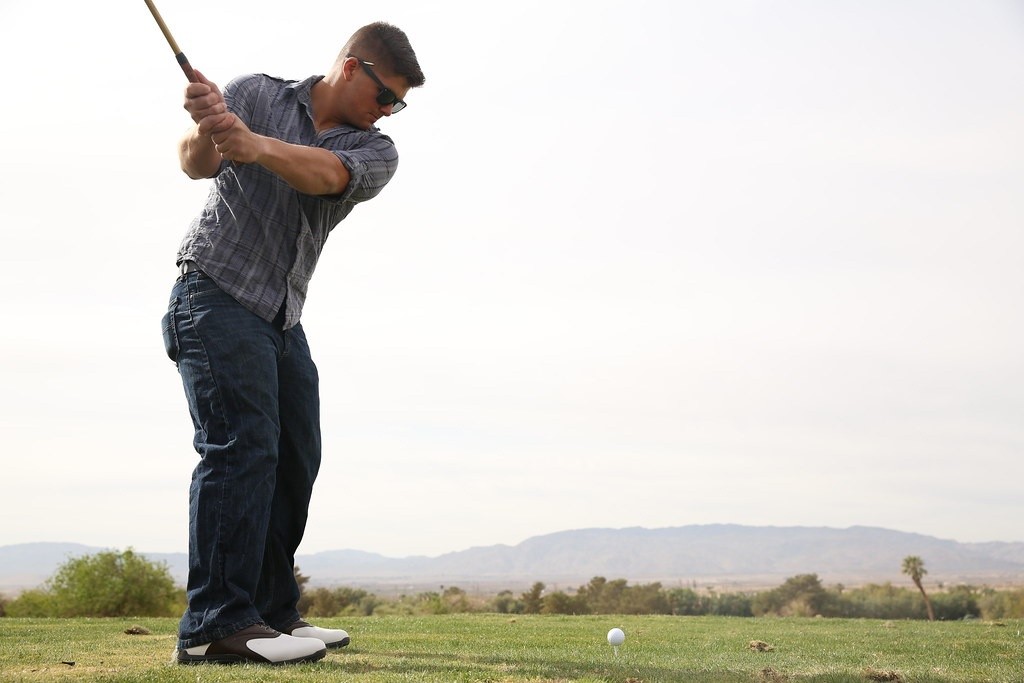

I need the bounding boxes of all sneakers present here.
[177,622,325,666]
[271,621,350,649]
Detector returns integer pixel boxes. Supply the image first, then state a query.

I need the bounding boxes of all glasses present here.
[344,54,407,114]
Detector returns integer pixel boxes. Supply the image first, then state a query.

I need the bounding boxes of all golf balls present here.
[608,629,624,647]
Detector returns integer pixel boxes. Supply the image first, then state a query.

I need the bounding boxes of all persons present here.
[160,21,425,665]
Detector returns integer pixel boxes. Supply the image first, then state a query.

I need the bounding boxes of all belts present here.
[179,261,201,276]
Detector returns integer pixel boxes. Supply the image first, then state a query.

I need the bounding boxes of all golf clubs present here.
[145,0,197,82]
